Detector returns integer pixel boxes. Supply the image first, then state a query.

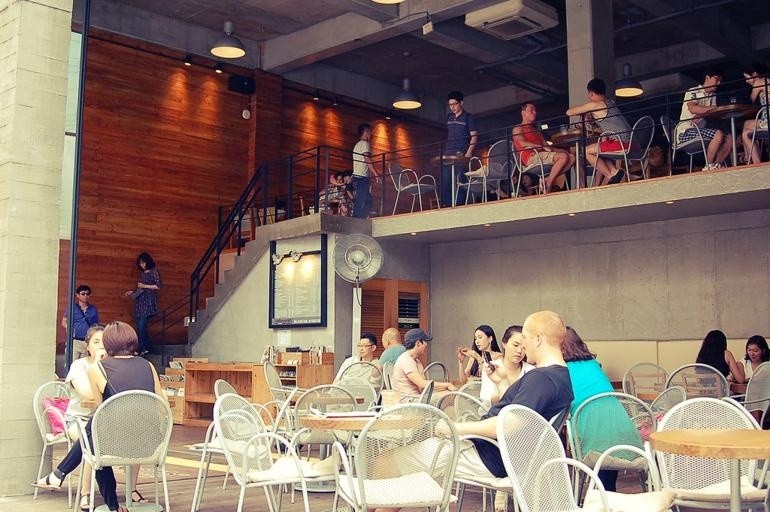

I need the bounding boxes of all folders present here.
[271,346,273,361]
[326,412,375,418]
[265,346,270,362]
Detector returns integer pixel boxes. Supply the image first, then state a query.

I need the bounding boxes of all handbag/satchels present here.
[128,287,144,301]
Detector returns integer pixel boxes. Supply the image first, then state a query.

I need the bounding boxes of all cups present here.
[379,390,403,417]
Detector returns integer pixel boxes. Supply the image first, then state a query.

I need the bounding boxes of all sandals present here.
[80,492,95,512]
[31,471,64,492]
[124,490,148,503]
[113,507,128,512]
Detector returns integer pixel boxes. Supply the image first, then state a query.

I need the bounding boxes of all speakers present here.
[228,74,256,94]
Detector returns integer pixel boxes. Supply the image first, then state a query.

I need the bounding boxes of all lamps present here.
[211,19,247,59]
[391,51,424,110]
[612,62,645,99]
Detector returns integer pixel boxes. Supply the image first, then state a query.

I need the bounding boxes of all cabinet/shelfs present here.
[157,358,208,425]
[359,277,431,378]
[182,361,272,428]
[264,348,336,413]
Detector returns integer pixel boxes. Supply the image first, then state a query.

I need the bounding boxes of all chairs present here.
[648,398,770,510]
[362,383,434,483]
[188,376,275,512]
[250,401,298,512]
[659,112,710,175]
[211,392,311,512]
[425,362,445,388]
[31,378,85,512]
[425,404,573,512]
[462,140,519,203]
[283,385,357,512]
[588,116,654,187]
[192,388,295,512]
[509,134,570,200]
[71,388,174,512]
[743,102,770,165]
[331,402,461,512]
[283,387,365,494]
[384,161,441,216]
[329,362,383,446]
[380,361,398,394]
[740,362,770,413]
[642,388,684,453]
[455,163,502,207]
[565,390,659,489]
[61,312,90,364]
[455,381,487,421]
[663,363,725,400]
[620,365,673,415]
[494,403,673,512]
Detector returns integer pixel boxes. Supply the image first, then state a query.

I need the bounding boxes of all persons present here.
[739,66,770,163]
[370,310,575,512]
[61,286,98,362]
[439,90,478,207]
[391,329,458,403]
[676,66,734,171]
[495,175,522,197]
[694,329,745,397]
[318,169,355,216]
[728,335,769,385]
[351,123,382,217]
[512,102,575,194]
[331,332,381,393]
[560,326,645,491]
[521,174,536,194]
[565,78,634,185]
[65,324,150,511]
[478,325,535,414]
[455,324,502,385]
[371,327,407,377]
[124,252,162,356]
[31,320,168,511]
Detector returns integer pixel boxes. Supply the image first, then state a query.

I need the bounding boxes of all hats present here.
[404,328,433,349]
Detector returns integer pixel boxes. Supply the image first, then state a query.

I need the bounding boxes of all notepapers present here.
[279,330,292,346]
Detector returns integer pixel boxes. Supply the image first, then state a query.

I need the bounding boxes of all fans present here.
[330,232,386,285]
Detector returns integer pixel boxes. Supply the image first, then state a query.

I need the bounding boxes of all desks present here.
[637,386,728,410]
[78,396,180,417]
[429,153,475,207]
[648,430,770,512]
[703,102,760,166]
[550,127,602,188]
[300,408,429,512]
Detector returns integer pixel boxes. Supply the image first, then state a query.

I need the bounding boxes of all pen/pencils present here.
[474,379,482,381]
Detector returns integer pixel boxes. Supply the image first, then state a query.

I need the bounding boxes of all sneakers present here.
[607,168,624,186]
[322,505,352,512]
[703,163,716,171]
[714,163,725,169]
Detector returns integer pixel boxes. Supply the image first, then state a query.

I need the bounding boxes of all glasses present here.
[80,291,90,296]
[448,102,459,106]
[357,344,373,348]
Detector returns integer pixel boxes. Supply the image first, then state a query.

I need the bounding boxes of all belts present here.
[73,338,85,341]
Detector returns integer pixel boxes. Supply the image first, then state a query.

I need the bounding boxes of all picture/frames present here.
[267,237,328,330]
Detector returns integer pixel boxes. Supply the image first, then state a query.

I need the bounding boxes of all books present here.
[166,386,184,397]
[159,374,183,382]
[169,361,182,369]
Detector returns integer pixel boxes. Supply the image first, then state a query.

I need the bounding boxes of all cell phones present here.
[483,350,494,371]
